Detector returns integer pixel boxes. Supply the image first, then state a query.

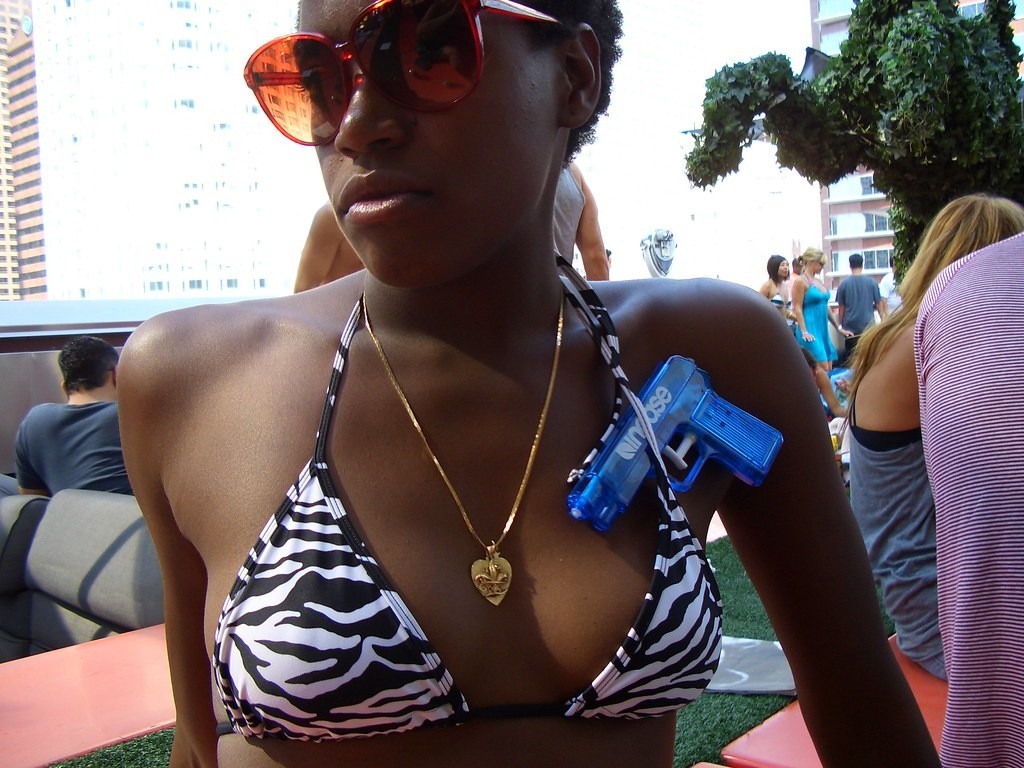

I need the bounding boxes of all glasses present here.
[814,260,825,266]
[243,1,571,146]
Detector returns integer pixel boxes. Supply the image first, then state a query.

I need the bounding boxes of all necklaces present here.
[361,283,567,607]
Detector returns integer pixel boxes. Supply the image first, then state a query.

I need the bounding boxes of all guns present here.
[566,356,784,533]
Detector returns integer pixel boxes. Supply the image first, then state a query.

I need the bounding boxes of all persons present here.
[16,335,134,497]
[292,160,886,417]
[848,193,1024,681]
[117,0,942,767]
[915,230,1024,768]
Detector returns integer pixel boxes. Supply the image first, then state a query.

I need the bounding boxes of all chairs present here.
[0,487,164,663]
